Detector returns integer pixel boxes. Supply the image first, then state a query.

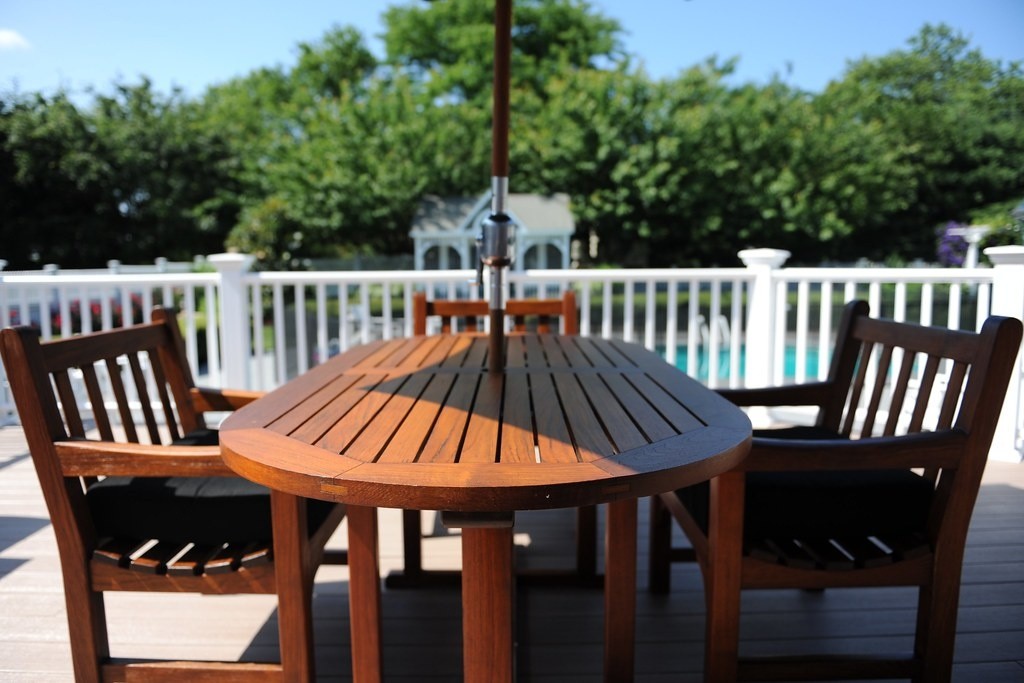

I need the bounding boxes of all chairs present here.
[1,306,347,683]
[646,298,1024,683]
[385,291,607,595]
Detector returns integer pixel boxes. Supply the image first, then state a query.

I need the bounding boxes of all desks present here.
[217,330,752,682]
